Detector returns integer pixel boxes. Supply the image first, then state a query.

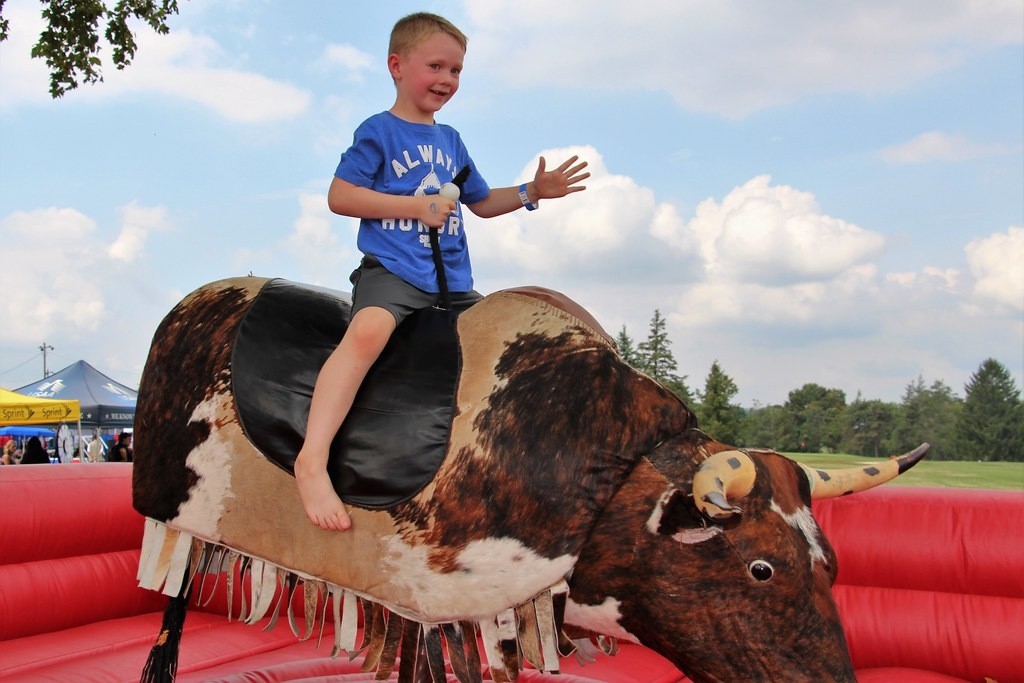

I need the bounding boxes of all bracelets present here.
[518,184,539,211]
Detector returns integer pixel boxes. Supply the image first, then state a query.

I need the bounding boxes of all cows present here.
[129,272,931,683]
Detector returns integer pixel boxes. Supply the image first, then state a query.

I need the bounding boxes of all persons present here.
[19,435,51,464]
[110,431,132,462]
[291,11,592,532]
[0,440,17,465]
[87,434,103,463]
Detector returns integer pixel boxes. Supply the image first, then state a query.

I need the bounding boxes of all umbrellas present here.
[0,424,56,455]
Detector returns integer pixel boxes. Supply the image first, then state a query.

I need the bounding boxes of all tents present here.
[11,359,139,466]
[0,387,83,464]
